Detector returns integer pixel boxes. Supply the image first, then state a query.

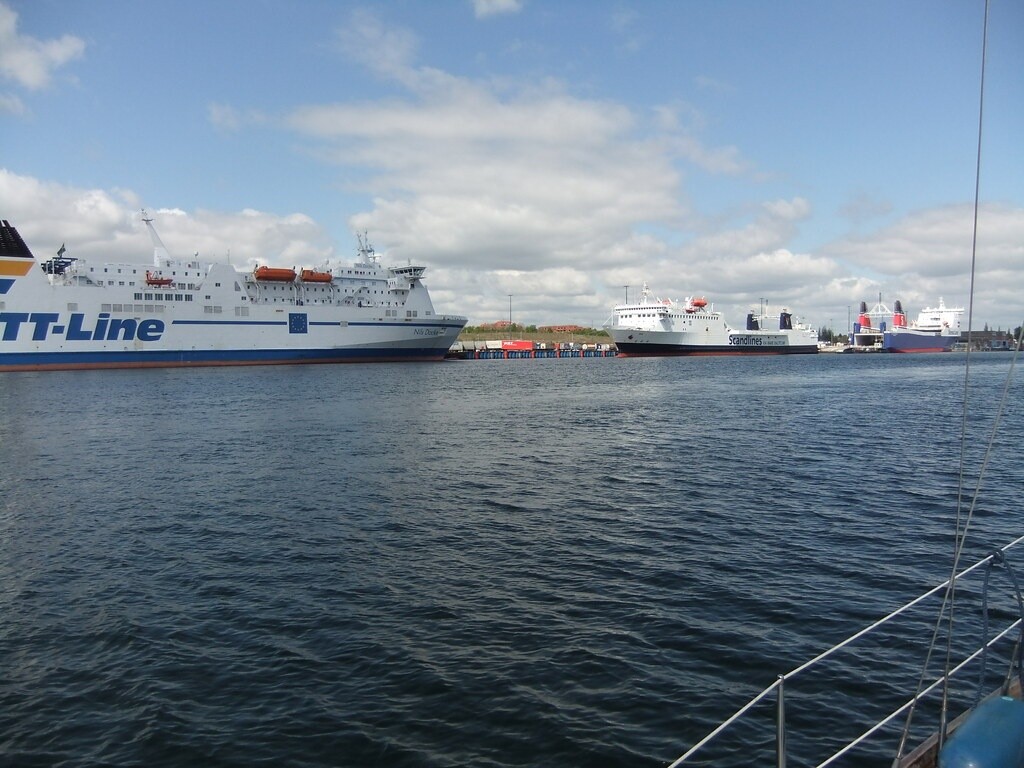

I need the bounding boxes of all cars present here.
[609,347,617,351]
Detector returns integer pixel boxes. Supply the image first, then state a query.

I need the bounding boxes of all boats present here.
[848,292,965,353]
[299,270,333,283]
[836,348,854,353]
[985,340,1009,351]
[685,308,694,314]
[254,266,295,282]
[0,208,469,372]
[952,343,972,352]
[691,298,707,307]
[146,271,173,285]
[601,281,818,356]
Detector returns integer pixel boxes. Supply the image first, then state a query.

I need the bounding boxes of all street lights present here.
[508,294,513,339]
[760,298,764,330]
[624,286,629,304]
[848,306,851,332]
